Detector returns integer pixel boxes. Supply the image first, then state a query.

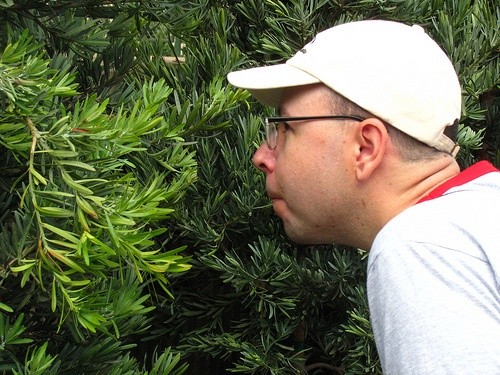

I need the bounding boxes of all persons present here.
[227,20,500,375]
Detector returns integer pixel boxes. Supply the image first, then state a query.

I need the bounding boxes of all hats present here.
[228,21,461,157]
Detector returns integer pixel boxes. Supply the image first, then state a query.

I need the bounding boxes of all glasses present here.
[265,114,365,148]
